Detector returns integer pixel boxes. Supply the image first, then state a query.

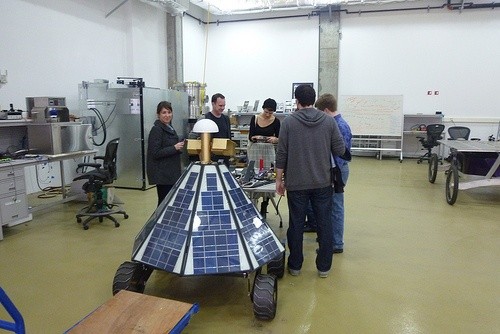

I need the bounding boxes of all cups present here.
[21,111,28,119]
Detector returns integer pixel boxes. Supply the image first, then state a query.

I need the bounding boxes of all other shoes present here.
[318,269,328,277]
[304,224,318,233]
[286,262,301,276]
[316,246,343,254]
[304,221,310,226]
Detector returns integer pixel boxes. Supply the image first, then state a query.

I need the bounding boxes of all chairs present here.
[73,138,129,230]
[416,124,470,165]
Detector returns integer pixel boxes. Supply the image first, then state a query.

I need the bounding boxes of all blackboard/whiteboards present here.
[337,95,404,137]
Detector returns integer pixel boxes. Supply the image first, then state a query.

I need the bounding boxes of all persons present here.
[247,98,280,218]
[315,94,351,253]
[275,84,345,278]
[147,101,184,208]
[188,93,230,169]
[293,107,316,232]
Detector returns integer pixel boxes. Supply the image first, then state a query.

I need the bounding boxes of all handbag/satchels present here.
[332,166,344,193]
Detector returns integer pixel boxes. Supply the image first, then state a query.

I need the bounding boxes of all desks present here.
[232,168,285,228]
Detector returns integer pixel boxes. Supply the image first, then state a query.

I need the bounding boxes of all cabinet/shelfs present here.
[231,112,292,157]
[0,119,98,241]
[401,114,442,160]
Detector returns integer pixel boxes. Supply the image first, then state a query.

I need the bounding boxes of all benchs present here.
[350,134,402,160]
